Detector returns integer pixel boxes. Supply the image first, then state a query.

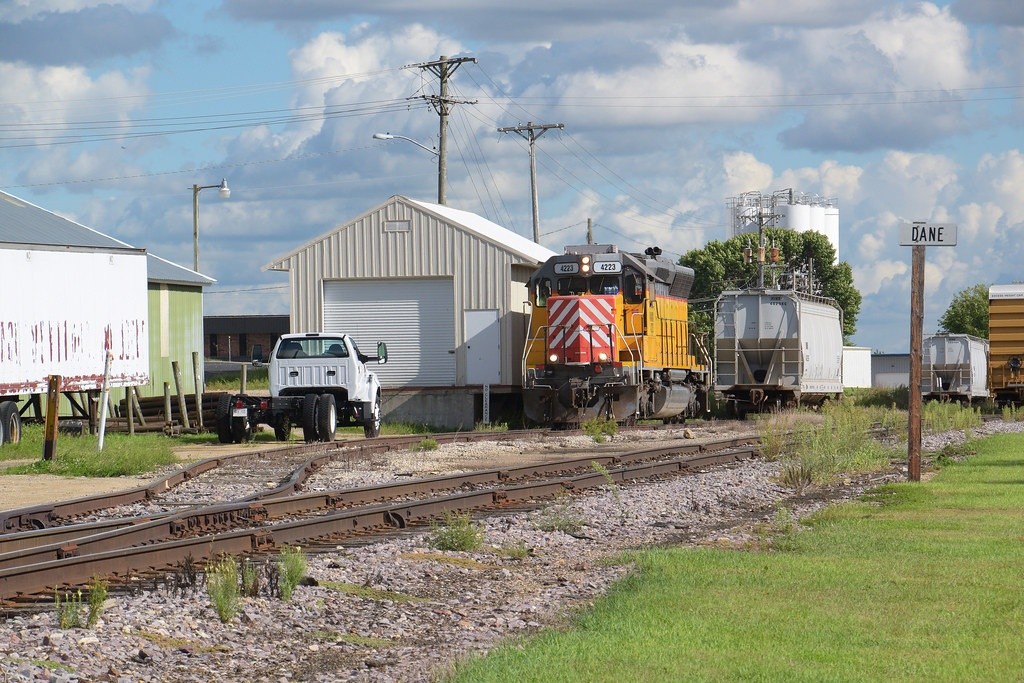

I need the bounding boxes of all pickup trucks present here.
[217,332,388,443]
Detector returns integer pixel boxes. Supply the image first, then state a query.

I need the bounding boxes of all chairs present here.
[280,343,307,357]
[327,344,343,355]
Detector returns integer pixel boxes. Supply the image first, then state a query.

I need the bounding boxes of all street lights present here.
[187,177,230,274]
[372,131,447,205]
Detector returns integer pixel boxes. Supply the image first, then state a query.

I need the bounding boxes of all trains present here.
[520,242,845,428]
